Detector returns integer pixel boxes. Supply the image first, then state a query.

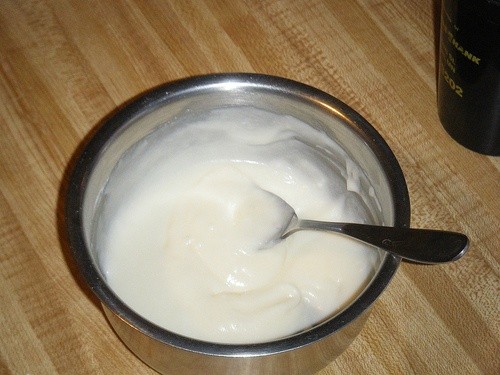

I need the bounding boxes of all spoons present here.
[231,187,470,264]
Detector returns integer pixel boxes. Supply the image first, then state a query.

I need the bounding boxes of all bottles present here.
[436,0,499,162]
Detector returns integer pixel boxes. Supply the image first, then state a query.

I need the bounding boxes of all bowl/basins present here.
[63,72,411,375]
